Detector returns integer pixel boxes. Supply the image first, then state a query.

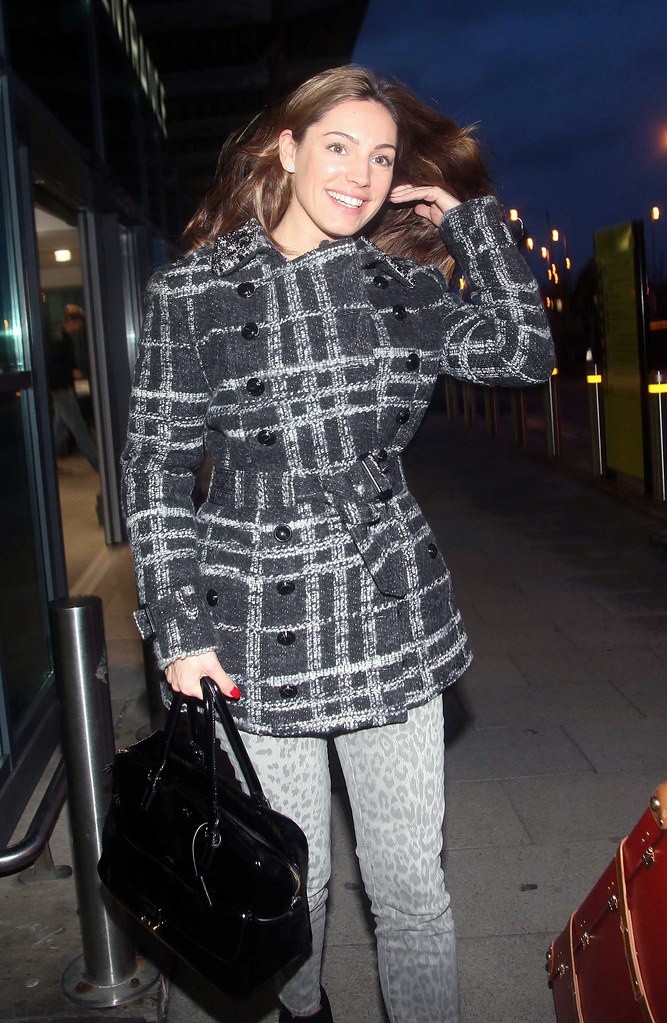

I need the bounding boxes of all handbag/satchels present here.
[97,677,313,1000]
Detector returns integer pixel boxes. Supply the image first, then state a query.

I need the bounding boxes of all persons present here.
[44,302,99,473]
[120,64,555,1023]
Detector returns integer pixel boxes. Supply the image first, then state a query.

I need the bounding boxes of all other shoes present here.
[279,984,333,1023]
